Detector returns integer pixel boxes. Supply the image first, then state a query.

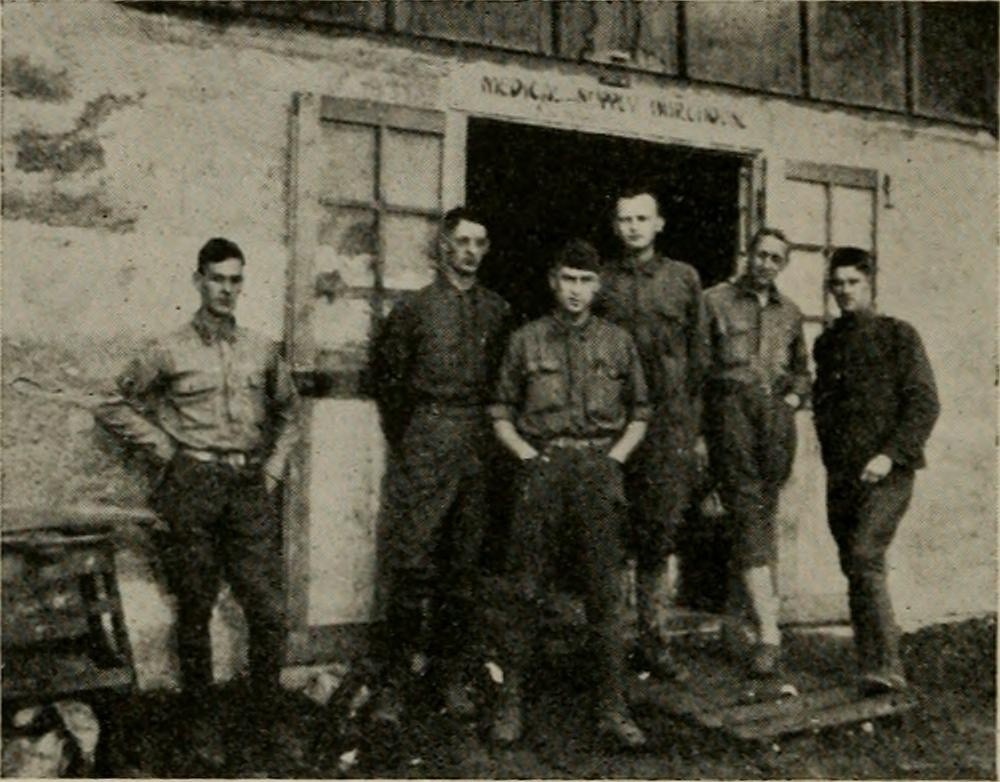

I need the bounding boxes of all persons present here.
[813,246,941,692]
[369,208,511,726]
[484,238,648,747]
[702,227,812,672]
[95,236,306,709]
[591,188,711,676]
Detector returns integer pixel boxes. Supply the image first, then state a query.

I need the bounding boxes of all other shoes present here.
[437,685,479,732]
[251,703,302,758]
[367,677,407,726]
[595,703,648,753]
[632,643,690,683]
[484,688,524,745]
[846,646,910,693]
[177,706,226,769]
[725,619,785,676]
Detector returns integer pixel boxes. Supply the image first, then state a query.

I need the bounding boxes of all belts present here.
[528,435,614,451]
[178,442,263,469]
